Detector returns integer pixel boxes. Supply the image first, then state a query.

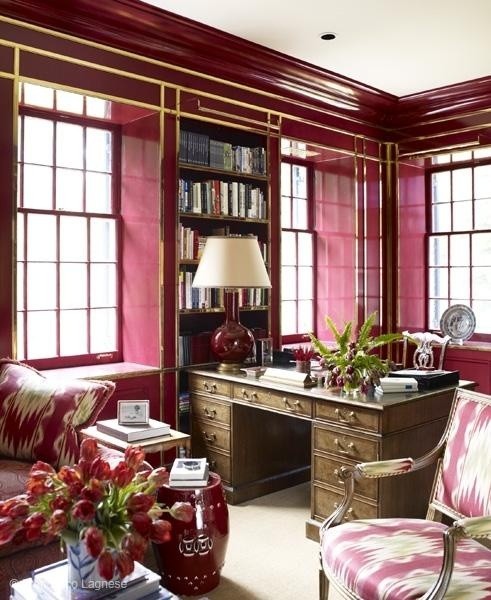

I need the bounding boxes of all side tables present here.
[78,425,191,467]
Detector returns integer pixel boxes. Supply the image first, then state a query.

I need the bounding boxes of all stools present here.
[152,471,229,597]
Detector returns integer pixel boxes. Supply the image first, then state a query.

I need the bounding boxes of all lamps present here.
[192,236,273,370]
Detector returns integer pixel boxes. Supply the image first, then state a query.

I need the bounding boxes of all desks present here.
[184,360,479,543]
[8,558,181,600]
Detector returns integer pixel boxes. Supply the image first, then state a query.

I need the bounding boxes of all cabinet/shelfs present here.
[121,110,281,429]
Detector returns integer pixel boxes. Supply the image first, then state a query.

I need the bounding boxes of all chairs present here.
[402,329,452,371]
[319,387,490,599]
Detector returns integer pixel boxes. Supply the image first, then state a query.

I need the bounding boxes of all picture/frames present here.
[117,400,150,425]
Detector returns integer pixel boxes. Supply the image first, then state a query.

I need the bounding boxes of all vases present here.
[66,543,122,600]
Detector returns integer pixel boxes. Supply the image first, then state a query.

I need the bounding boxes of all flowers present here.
[305,311,422,395]
[0,437,194,581]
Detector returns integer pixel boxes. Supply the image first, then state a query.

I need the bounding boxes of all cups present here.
[261,338,273,366]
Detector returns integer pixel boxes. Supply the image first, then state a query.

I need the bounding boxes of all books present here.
[169,457,207,480]
[177,130,267,414]
[169,463,210,487]
[31,559,173,600]
[96,418,171,442]
[379,377,419,393]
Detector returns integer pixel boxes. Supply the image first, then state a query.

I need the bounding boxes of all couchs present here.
[0,456,57,558]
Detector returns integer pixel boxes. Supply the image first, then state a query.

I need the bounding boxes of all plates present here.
[439,304,476,341]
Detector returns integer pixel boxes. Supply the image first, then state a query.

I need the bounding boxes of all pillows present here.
[0,358,116,469]
